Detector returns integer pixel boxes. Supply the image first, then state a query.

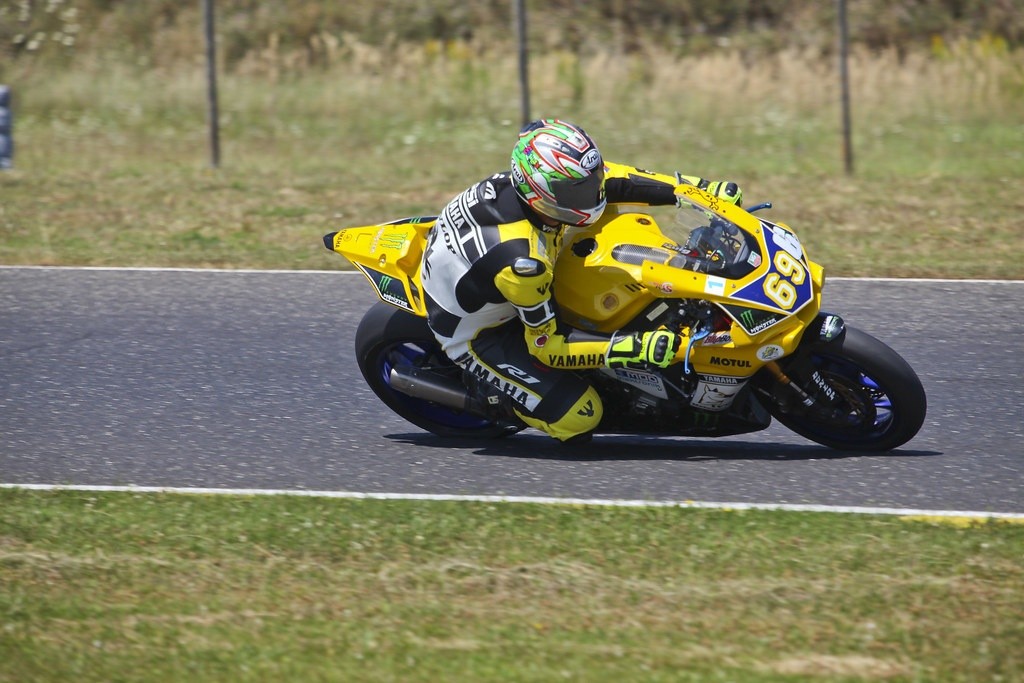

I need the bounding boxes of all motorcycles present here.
[322,183,926,454]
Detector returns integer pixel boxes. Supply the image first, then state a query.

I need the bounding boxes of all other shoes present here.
[472,374,522,427]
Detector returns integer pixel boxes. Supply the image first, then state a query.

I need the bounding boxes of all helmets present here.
[510,118,607,228]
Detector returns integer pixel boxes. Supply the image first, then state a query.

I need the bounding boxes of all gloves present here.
[676,172,743,219]
[605,330,681,370]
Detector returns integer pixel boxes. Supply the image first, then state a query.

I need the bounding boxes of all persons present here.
[423,118,743,445]
